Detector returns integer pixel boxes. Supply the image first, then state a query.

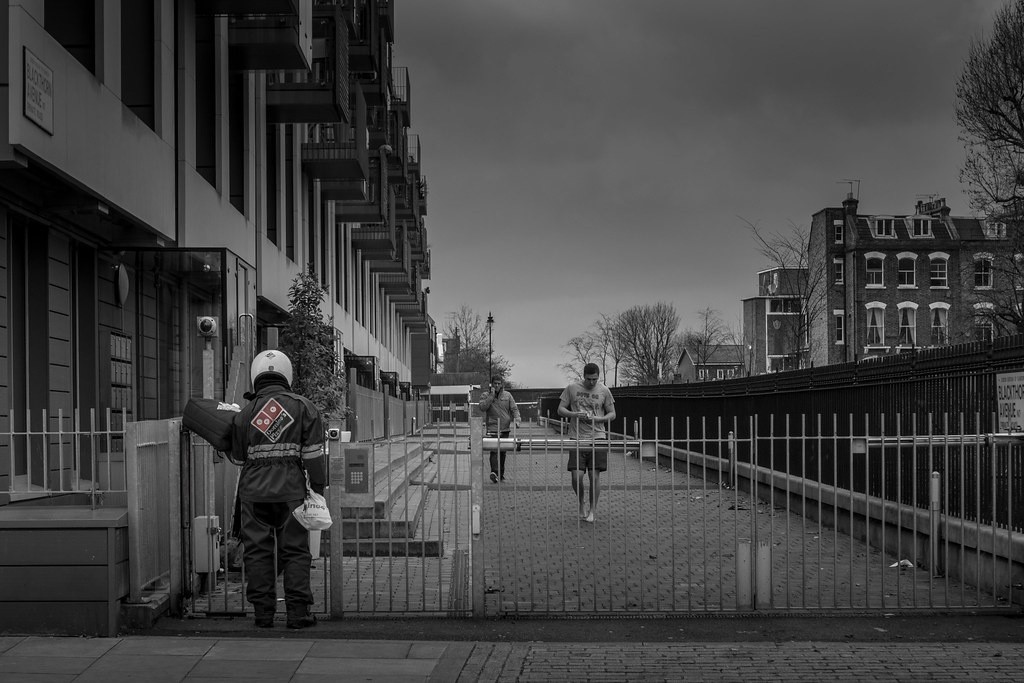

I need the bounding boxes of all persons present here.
[479,375,521,483]
[223,348,327,629]
[556,363,616,523]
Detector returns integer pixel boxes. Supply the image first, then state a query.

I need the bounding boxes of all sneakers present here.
[287,612,317,629]
[254,619,274,628]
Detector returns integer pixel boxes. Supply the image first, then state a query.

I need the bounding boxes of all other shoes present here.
[500,476,506,481]
[490,472,498,484]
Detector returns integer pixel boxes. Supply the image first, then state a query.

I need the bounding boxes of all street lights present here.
[486,311,494,434]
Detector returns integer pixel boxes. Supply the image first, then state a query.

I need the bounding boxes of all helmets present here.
[251,350,293,393]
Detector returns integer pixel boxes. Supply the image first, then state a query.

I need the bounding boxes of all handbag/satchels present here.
[292,470,333,532]
[182,398,241,452]
